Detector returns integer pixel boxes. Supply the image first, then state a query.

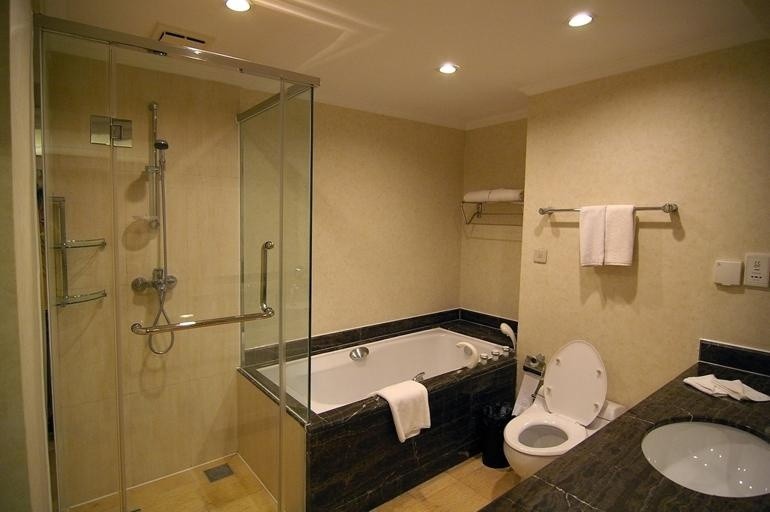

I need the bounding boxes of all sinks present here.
[640,415,770,499]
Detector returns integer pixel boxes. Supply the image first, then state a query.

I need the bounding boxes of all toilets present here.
[503,340,626,482]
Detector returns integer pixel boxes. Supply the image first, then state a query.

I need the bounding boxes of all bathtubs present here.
[254,326,512,415]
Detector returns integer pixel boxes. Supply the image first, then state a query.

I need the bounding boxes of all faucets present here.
[456,342,479,368]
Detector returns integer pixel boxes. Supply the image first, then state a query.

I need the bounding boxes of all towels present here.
[577,202,605,268]
[682,371,729,398]
[602,205,640,267]
[365,378,433,446]
[489,186,523,201]
[714,375,770,405]
[462,190,493,202]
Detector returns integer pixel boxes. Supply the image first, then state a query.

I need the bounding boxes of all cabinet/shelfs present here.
[49,239,109,307]
